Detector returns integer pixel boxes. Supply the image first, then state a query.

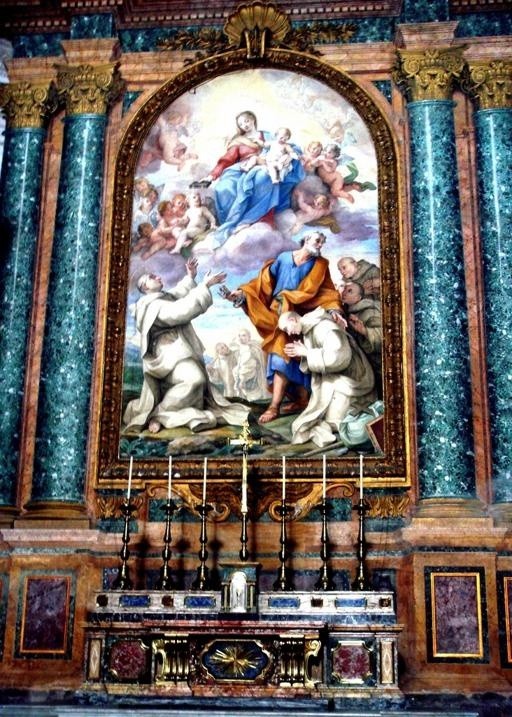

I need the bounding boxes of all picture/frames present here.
[423,565,490,664]
[495,570,512,668]
[12,569,77,660]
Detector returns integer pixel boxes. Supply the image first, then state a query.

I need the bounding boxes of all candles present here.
[114,454,367,513]
